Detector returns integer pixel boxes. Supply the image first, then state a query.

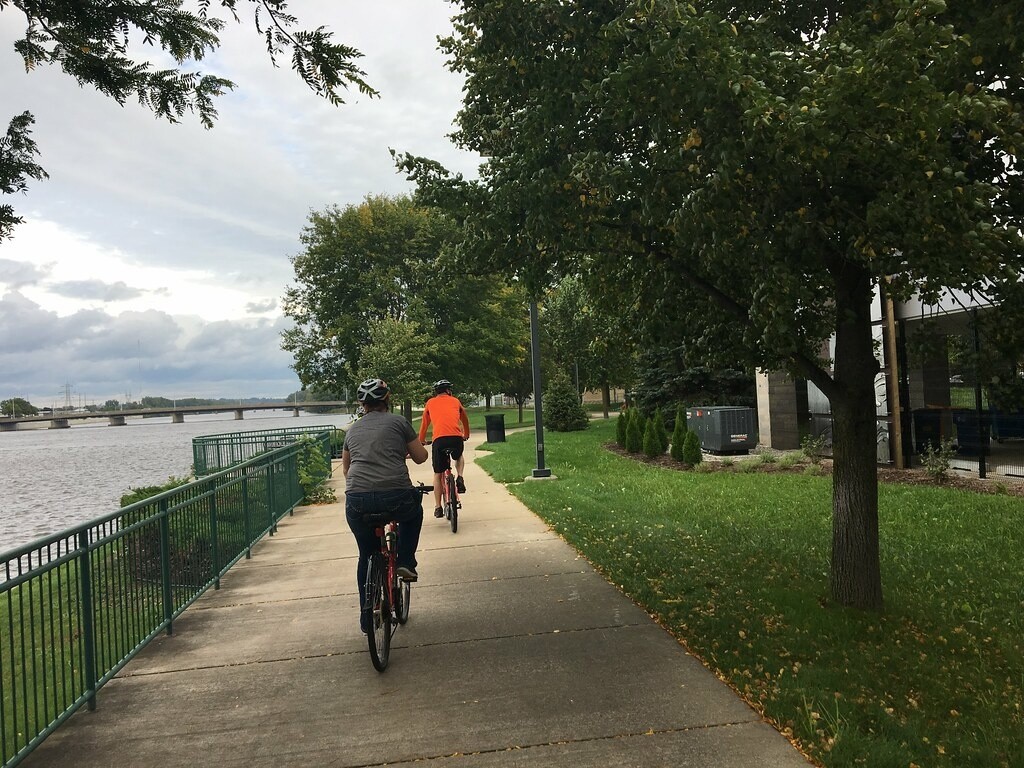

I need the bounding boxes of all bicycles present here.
[422,437,470,534]
[361,480,435,673]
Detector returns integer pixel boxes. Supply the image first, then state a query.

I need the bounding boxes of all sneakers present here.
[456,476,466,494]
[434,507,443,518]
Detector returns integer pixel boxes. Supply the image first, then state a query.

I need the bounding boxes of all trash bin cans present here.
[991,413,1024,444]
[685,405,758,456]
[484,414,506,443]
[952,407,994,457]
[911,408,944,456]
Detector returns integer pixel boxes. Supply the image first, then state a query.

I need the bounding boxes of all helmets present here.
[357,378,390,403]
[433,379,453,392]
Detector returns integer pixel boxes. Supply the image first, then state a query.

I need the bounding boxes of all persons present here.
[343,378,428,633]
[419,379,469,518]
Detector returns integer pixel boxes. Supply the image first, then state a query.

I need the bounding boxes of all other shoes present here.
[396,559,417,577]
[360,615,380,632]
